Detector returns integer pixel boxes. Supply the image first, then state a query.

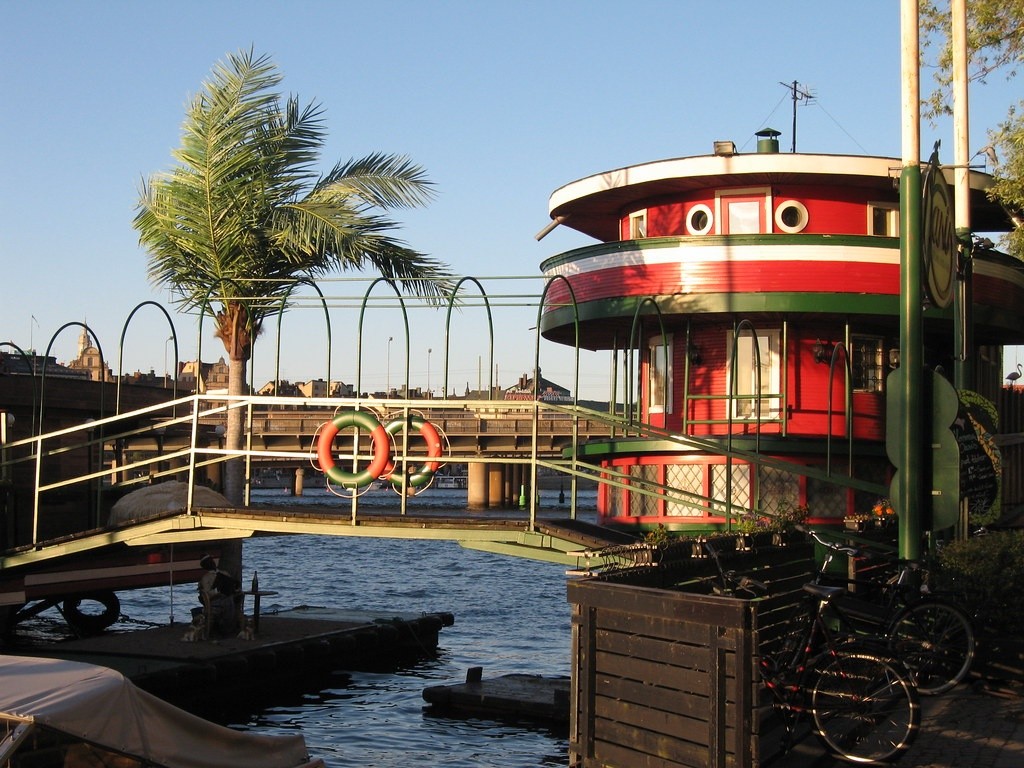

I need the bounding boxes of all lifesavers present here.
[63,590,121,629]
[309,406,397,498]
[370,410,451,497]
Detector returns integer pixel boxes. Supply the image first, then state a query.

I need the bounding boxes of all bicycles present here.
[760,522,978,698]
[681,542,921,768]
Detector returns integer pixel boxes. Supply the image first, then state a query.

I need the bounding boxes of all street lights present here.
[428,348,431,400]
[387,337,394,418]
[164,336,175,388]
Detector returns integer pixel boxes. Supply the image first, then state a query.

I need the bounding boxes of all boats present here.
[0,656,324,768]
